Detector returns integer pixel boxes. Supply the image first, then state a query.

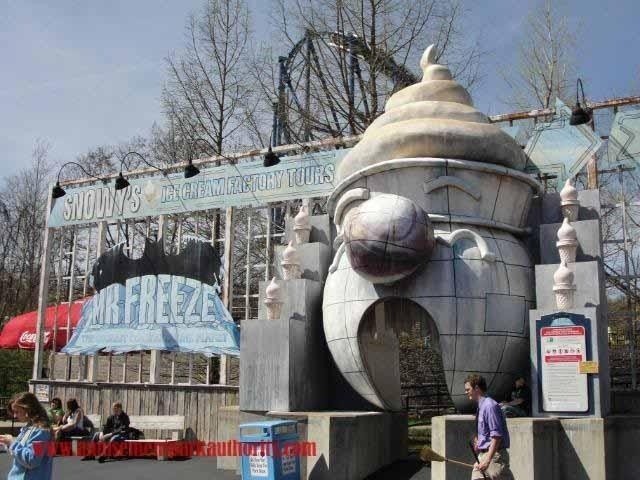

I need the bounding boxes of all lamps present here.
[52,139,236,198]
[262,120,311,167]
[568,77,593,125]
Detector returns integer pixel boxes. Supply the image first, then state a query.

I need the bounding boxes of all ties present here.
[475,407,480,440]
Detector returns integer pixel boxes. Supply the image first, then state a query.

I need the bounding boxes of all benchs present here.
[51,413,187,460]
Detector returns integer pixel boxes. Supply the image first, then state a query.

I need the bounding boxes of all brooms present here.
[420,445,473,468]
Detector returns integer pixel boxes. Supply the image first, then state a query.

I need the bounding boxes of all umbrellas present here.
[0,295,93,352]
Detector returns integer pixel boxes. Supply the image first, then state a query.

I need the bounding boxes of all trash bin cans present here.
[237,421,300,480]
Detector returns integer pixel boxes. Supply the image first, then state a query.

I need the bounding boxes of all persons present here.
[81,400,130,462]
[0,392,54,480]
[496,376,532,418]
[46,397,64,429]
[52,398,84,450]
[463,373,516,479]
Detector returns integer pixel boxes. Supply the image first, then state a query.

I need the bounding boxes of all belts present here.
[478,449,488,453]
